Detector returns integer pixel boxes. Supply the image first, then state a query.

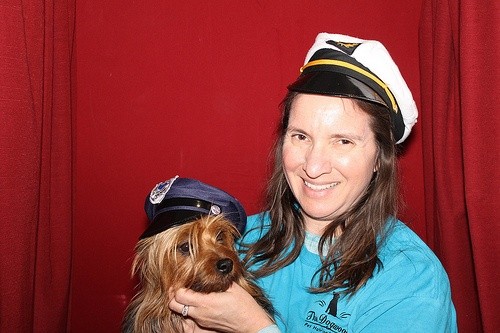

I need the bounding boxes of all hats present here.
[138,175,249,242]
[286,32,419,145]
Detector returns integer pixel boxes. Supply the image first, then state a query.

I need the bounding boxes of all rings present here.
[181,305,189,317]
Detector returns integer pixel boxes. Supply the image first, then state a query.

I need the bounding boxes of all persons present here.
[167,32,458,333]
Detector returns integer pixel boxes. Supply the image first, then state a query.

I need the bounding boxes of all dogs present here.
[124,175,278,333]
